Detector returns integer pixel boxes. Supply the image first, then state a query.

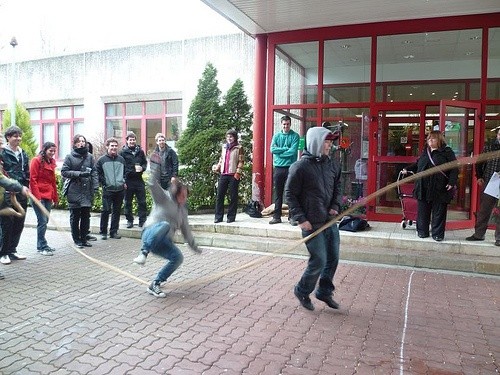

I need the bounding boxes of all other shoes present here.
[268,217,282,224]
[100,231,120,240]
[127,220,145,228]
[297,291,314,310]
[85,235,98,241]
[467,235,484,242]
[214,218,223,223]
[417,232,443,241]
[226,219,234,223]
[494,239,500,246]
[36,245,55,256]
[315,290,340,310]
[288,218,299,226]
[77,239,92,248]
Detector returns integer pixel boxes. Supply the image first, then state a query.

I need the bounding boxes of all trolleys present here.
[397,170,420,228]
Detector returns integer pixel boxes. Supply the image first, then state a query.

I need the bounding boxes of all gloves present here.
[80,171,90,178]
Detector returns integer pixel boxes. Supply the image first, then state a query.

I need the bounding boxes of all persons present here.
[285,126,344,312]
[150,133,179,191]
[400,130,458,241]
[118,133,147,229]
[465,127,500,246]
[97,136,129,239]
[354,153,370,180]
[269,116,300,226]
[134,149,204,298]
[61,134,99,248]
[0,125,30,265]
[29,141,59,256]
[0,133,29,280]
[83,142,98,241]
[212,129,244,224]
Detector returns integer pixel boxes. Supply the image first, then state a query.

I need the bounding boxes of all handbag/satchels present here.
[448,184,458,200]
[62,178,71,197]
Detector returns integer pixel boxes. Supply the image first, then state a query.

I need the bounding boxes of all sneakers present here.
[146,281,167,298]
[1,252,26,264]
[133,253,146,265]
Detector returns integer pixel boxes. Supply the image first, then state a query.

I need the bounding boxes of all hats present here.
[225,128,238,137]
[324,132,339,141]
